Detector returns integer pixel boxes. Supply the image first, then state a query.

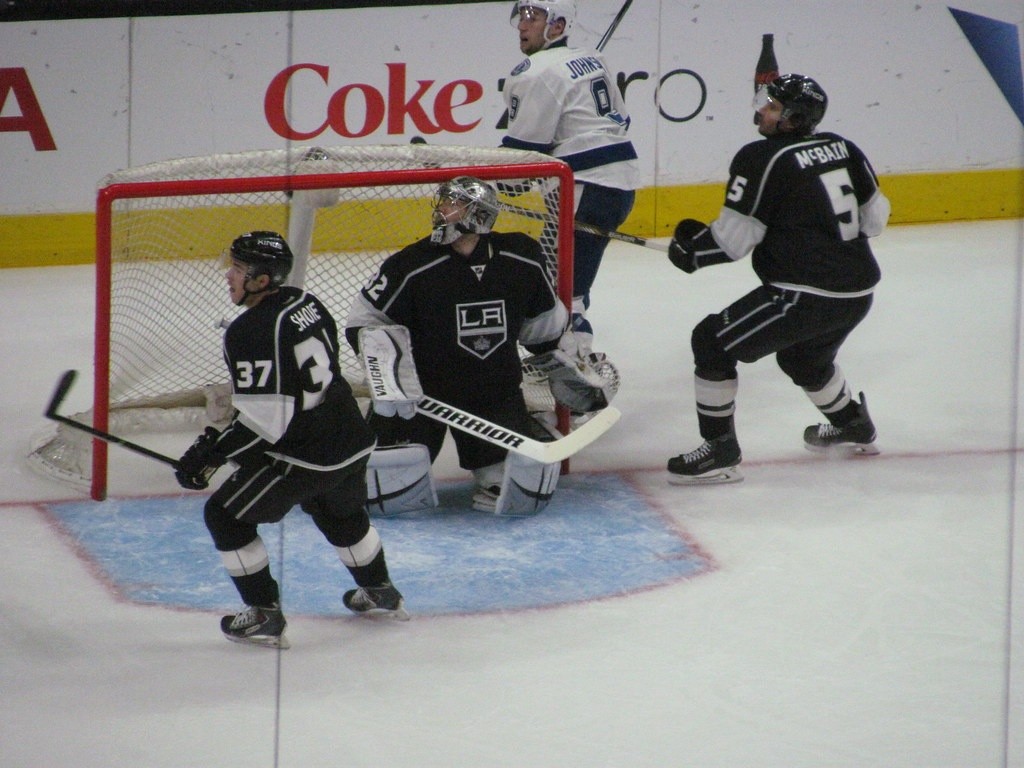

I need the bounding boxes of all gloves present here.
[670,219,707,273]
[178,426,226,489]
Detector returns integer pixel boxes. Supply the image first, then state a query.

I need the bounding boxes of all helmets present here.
[231,231,293,289]
[767,73,827,125]
[518,0,577,37]
[431,175,499,246]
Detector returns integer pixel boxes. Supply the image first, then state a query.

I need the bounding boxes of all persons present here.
[345,176,620,517]
[175,230,410,648]
[667,73,891,486]
[498,0,642,429]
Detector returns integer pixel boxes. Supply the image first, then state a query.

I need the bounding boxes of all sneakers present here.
[221,601,290,650]
[343,580,410,621]
[667,418,743,485]
[804,391,881,456]
[471,489,501,512]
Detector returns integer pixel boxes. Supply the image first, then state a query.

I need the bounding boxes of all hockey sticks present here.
[43,369,182,469]
[413,394,623,465]
[409,136,672,254]
[595,0,633,54]
